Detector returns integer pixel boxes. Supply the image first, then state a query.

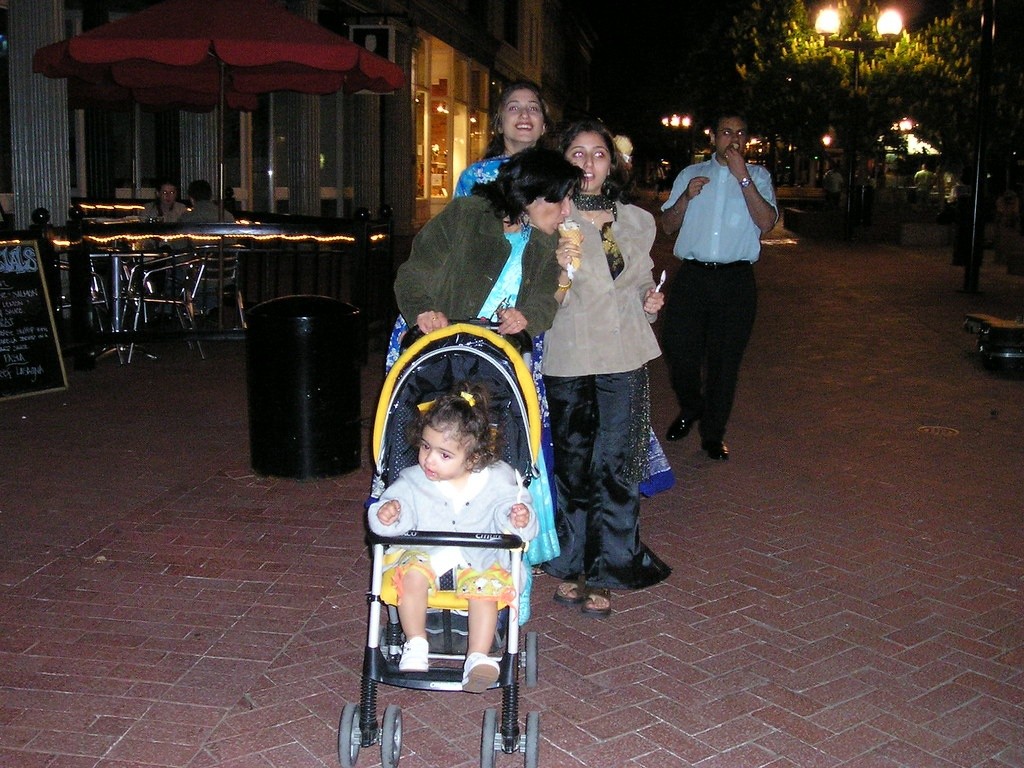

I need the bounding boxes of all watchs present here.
[739,178,753,187]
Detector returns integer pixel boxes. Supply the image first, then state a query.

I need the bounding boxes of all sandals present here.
[581,587,610,618]
[554,580,584,605]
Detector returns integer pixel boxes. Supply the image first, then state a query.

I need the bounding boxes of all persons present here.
[455,81,674,497]
[377,382,539,691]
[138,183,187,314]
[179,180,236,318]
[541,124,670,613]
[656,165,665,192]
[915,163,938,206]
[945,174,1019,266]
[367,145,589,513]
[822,163,885,237]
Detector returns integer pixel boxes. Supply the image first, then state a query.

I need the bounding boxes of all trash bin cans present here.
[243,293,360,481]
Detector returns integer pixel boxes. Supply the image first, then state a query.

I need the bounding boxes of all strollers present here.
[335,322,542,767]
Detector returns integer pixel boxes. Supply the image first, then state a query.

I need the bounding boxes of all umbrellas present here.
[32,0,407,329]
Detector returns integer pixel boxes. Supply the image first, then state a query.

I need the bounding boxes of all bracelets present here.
[558,279,572,292]
[671,207,685,216]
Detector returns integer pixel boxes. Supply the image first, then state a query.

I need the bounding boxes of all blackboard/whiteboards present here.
[0,239,69,401]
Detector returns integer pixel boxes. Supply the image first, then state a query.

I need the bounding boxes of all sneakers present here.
[462,652,501,694]
[399,637,429,672]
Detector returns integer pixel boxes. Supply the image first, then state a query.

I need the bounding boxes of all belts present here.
[690,260,729,268]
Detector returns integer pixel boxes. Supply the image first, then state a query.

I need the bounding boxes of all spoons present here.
[654,269,667,293]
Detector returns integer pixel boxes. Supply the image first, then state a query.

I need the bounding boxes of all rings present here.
[652,303,656,309]
[432,316,438,321]
[725,155,729,158]
[516,320,520,326]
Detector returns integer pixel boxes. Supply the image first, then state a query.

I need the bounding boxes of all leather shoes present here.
[701,439,730,461]
[666,413,700,440]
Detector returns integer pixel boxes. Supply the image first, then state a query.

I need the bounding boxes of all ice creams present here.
[559,220,580,270]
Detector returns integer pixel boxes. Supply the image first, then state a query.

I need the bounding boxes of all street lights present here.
[660,113,694,173]
[811,3,905,243]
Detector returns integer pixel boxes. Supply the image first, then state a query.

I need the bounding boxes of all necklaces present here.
[660,115,779,461]
[588,211,603,224]
